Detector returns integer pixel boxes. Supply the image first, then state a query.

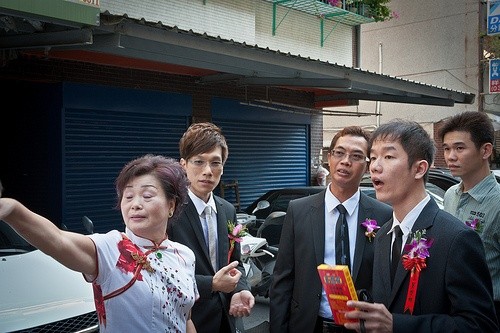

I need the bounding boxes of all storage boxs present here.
[317,263,359,325]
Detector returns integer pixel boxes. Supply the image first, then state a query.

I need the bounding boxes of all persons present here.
[269,127,393,333]
[167,123,255,333]
[438,112,500,333]
[344,119,499,333]
[0,155,200,333]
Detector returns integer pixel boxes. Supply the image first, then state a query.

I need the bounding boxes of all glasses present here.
[188,159,224,169]
[330,150,370,163]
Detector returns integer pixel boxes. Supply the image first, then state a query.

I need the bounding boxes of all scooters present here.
[236,199,287,306]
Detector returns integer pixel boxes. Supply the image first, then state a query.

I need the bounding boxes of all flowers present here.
[227,220,247,264]
[361,218,380,243]
[466,218,483,234]
[401,229,432,278]
[329,0,399,22]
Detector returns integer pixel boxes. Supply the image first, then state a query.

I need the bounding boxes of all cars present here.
[244,166,500,247]
[0,215,100,333]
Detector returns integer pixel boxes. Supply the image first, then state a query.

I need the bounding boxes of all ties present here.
[391,224,404,293]
[335,204,352,277]
[204,205,216,274]
